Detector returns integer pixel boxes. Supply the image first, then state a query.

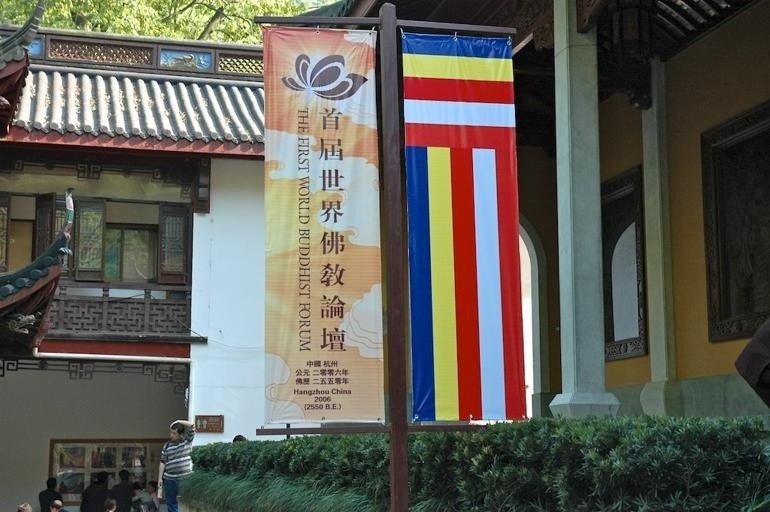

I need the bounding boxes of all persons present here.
[18,419,247,512]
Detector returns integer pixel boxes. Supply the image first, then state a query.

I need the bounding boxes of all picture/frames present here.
[589,166,646,364]
[49,438,172,505]
[700,100,769,344]
